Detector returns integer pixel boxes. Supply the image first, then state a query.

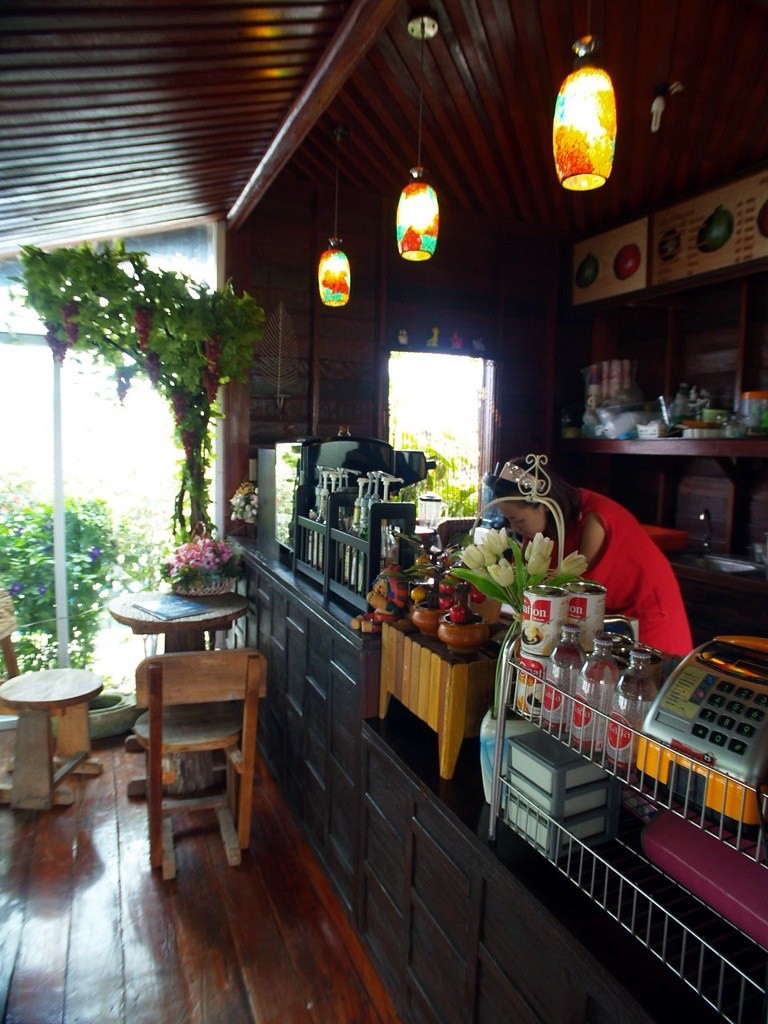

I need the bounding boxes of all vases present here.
[479,707,539,811]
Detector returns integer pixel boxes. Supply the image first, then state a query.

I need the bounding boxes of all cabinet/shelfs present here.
[293,484,415,615]
[555,257,768,546]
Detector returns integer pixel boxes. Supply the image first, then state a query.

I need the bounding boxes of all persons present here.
[492,454,694,662]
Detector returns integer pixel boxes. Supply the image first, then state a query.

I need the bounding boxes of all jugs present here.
[416,491,449,530]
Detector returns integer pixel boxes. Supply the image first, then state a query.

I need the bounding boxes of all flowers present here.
[451,528,588,719]
[158,536,243,590]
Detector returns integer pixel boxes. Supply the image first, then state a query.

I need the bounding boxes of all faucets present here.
[699,508,715,553]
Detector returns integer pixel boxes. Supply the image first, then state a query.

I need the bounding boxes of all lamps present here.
[318,122,351,307]
[396,13,440,262]
[551,0,618,191]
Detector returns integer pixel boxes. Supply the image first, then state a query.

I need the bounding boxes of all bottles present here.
[570,635,621,756]
[606,649,659,768]
[308,495,398,595]
[541,624,588,729]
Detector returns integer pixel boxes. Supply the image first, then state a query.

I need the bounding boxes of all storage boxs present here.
[503,729,623,861]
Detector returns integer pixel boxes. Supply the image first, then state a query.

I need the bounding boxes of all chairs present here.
[132,648,268,881]
[0,589,104,811]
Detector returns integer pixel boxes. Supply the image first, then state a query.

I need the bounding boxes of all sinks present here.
[668,550,766,575]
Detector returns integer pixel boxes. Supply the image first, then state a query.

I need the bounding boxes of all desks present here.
[109,590,248,798]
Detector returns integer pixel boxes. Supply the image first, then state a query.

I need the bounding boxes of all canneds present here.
[515,581,663,718]
